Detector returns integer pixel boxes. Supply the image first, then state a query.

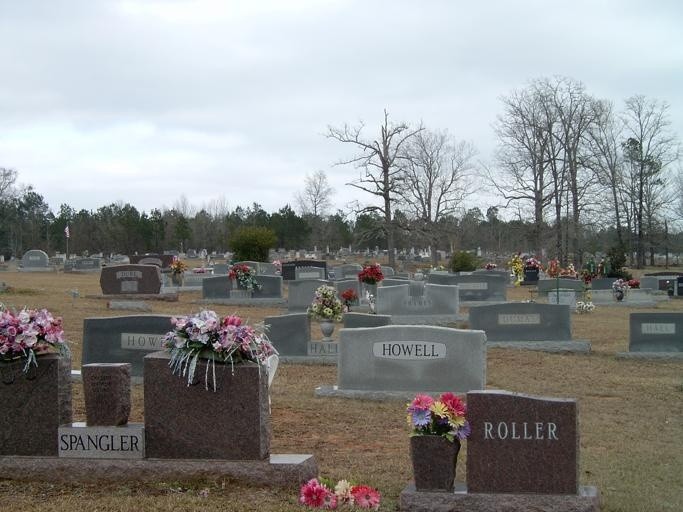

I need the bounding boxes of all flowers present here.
[306,285,343,321]
[0,306,70,374]
[399,392,471,442]
[159,310,273,395]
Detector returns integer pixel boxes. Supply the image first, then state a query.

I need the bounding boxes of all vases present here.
[344,300,352,312]
[409,435,460,491]
[183,346,242,363]
[0,342,62,360]
[322,319,335,341]
